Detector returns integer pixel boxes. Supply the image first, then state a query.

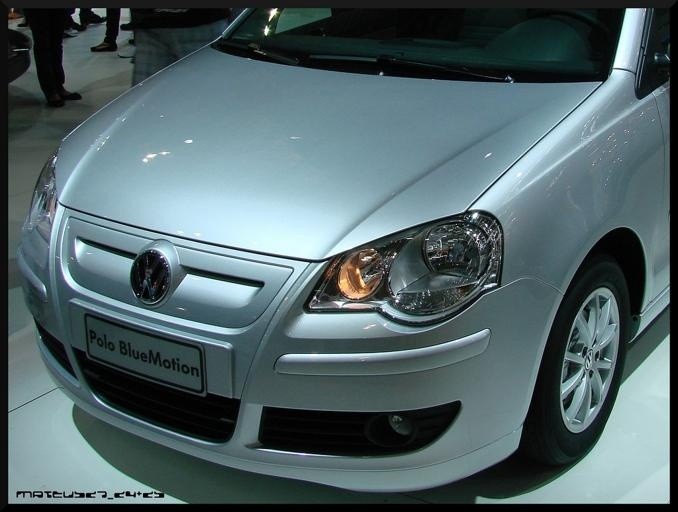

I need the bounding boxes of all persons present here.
[0,0,135,106]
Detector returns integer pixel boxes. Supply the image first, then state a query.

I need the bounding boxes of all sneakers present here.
[17,11,135,106]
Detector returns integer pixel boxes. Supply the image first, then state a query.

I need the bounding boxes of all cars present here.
[8,28,32,85]
[15,4,672,494]
